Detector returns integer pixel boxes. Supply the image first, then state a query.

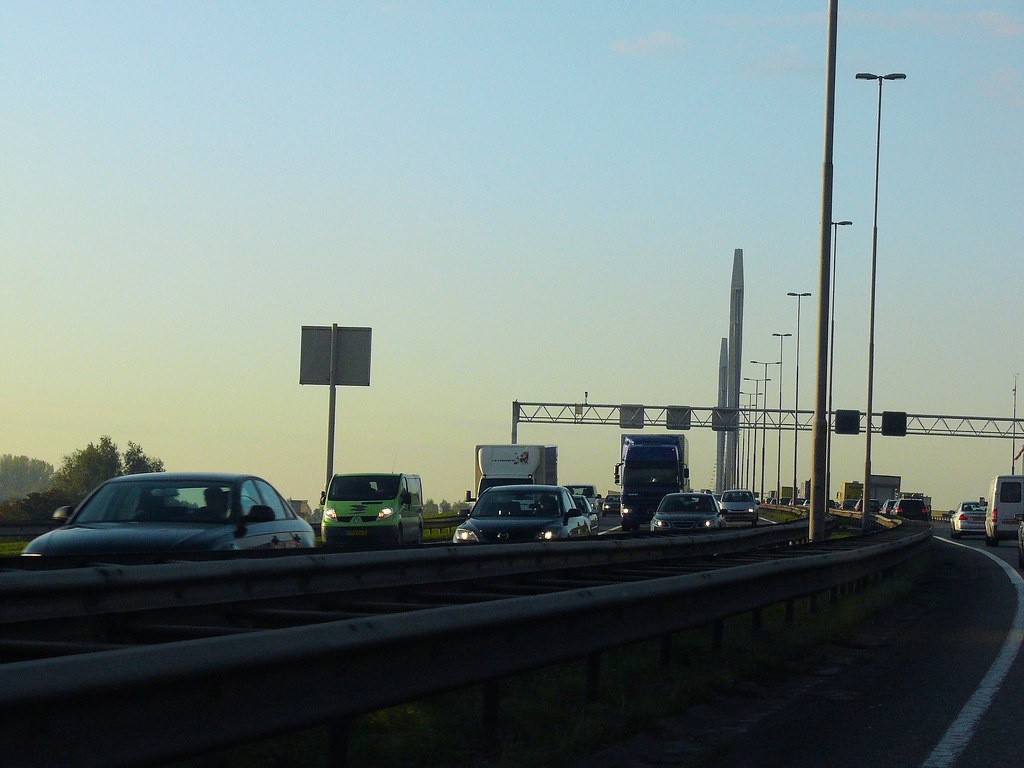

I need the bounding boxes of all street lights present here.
[855,72,907,530]
[786,292,812,508]
[744,378,771,499]
[772,334,792,505]
[740,392,763,490]
[739,404,756,490]
[750,361,782,504]
[820,221,853,513]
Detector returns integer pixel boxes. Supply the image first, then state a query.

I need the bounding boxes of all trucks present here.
[614,434,689,531]
[465,443,558,512]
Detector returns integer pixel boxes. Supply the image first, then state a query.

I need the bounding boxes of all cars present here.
[718,490,760,527]
[1014,514,1024,569]
[949,500,988,540]
[21,472,315,570]
[765,475,933,522]
[456,485,621,518]
[647,493,728,532]
[452,485,591,546]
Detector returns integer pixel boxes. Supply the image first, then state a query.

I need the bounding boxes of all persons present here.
[203,486,227,513]
[539,495,553,511]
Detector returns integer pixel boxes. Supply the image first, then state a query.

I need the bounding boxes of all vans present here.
[320,473,424,554]
[979,475,1024,548]
